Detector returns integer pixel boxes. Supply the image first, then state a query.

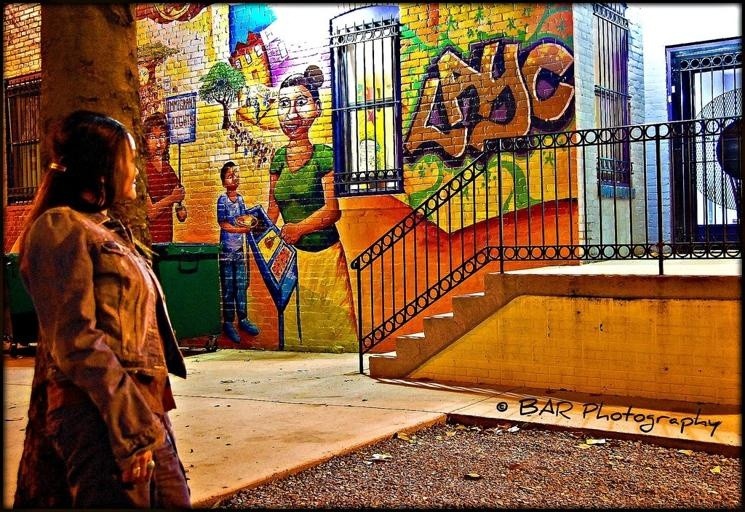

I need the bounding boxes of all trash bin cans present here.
[151,241,224,353]
[5,252,41,356]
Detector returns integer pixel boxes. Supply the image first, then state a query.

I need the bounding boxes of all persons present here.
[268,65,362,351]
[13,109,191,510]
[140,111,188,245]
[214,157,263,344]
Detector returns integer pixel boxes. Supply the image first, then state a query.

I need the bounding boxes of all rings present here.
[130,464,141,474]
[145,457,158,472]
